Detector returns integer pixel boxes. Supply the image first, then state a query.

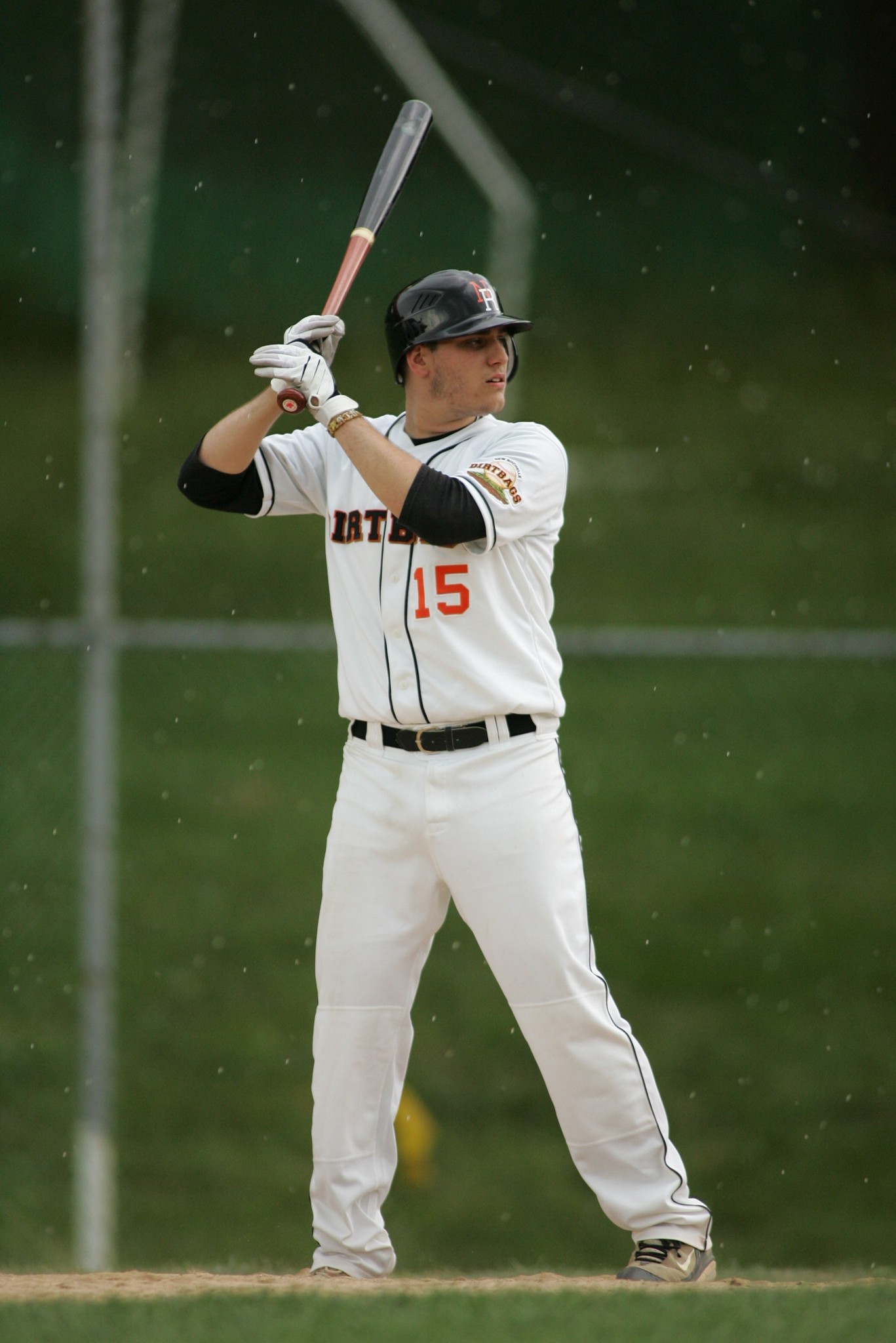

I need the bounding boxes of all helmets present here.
[385,270,534,386]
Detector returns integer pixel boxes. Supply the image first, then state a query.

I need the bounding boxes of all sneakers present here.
[616,1235,717,1285]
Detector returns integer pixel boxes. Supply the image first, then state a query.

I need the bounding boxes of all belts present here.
[350,714,536,754]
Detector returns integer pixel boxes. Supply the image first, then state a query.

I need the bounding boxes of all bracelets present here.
[325,409,364,438]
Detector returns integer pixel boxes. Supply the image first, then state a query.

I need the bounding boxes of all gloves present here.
[271,314,344,396]
[250,340,358,428]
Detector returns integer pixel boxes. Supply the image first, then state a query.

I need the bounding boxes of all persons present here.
[174,267,720,1286]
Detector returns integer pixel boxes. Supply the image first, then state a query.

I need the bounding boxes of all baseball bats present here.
[276,100,434,414]
